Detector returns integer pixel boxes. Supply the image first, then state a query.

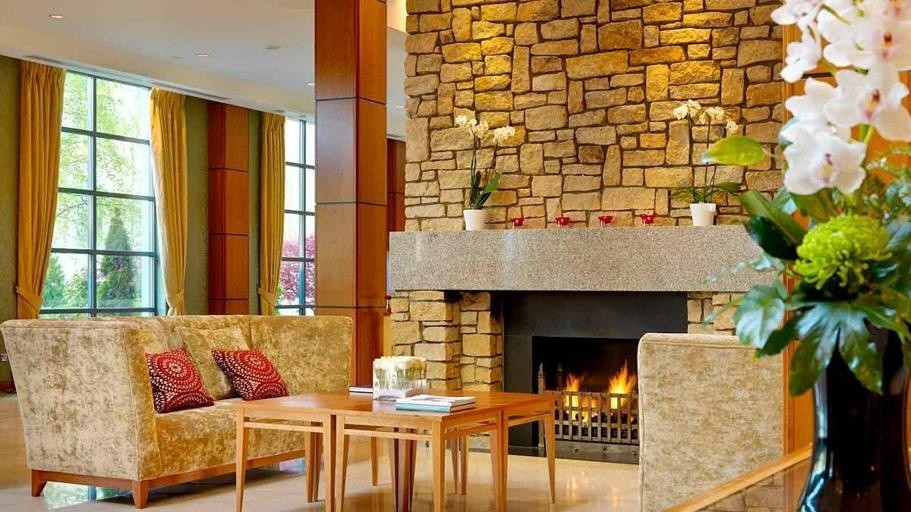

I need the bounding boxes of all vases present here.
[688,201,717,226]
[796,321,909,511]
[464,209,489,230]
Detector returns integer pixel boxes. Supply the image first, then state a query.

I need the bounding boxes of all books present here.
[394,394,476,413]
[348,384,373,393]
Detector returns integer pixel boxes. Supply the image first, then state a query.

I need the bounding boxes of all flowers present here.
[729,0,910,395]
[454,115,518,209]
[674,100,741,202]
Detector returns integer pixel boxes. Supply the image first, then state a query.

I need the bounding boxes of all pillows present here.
[144,326,290,415]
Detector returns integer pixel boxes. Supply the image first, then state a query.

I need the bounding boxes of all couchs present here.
[2,313,355,511]
